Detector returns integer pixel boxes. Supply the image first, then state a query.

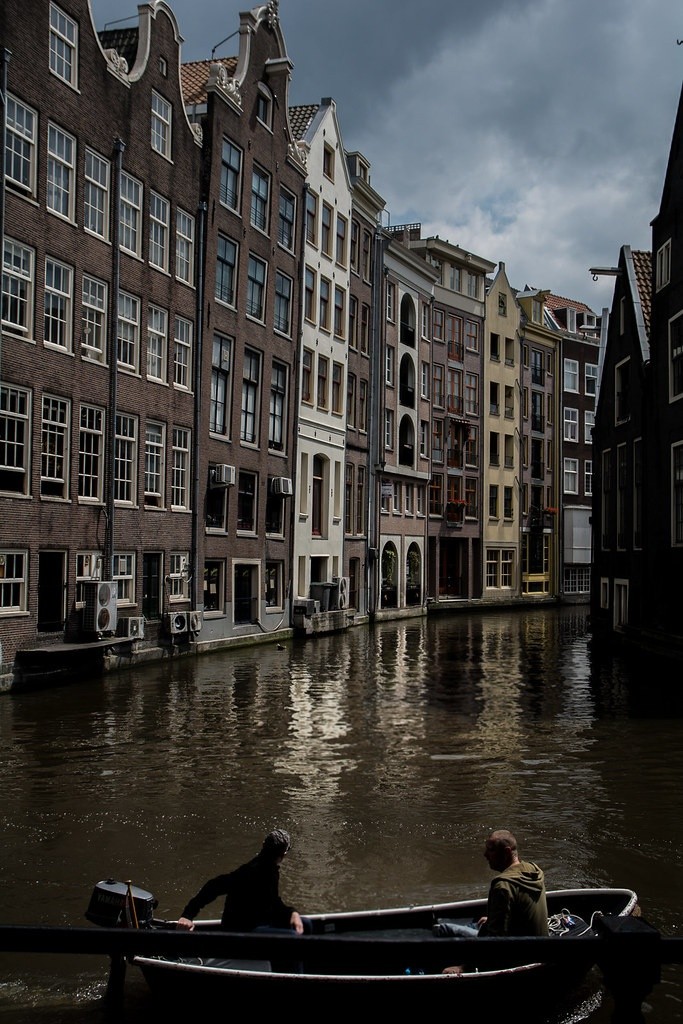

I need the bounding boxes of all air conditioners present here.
[188,610,203,634]
[83,581,118,634]
[273,477,294,497]
[217,463,235,484]
[121,617,144,641]
[333,577,350,610]
[166,611,187,634]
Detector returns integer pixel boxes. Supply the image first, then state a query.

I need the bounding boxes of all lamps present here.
[468,253,473,260]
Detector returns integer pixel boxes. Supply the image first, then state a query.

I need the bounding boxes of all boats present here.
[82,877,639,1024]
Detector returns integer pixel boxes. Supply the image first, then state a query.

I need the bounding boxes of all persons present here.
[176,829,312,974]
[433,830,549,936]
